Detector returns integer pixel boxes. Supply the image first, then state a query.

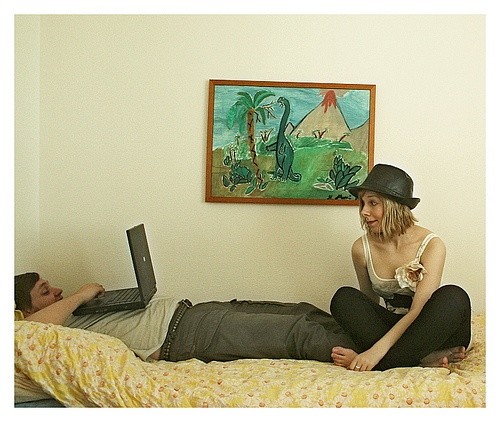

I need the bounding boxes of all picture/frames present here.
[204,79,376,206]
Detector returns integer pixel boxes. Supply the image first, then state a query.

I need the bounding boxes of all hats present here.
[349,164,420,209]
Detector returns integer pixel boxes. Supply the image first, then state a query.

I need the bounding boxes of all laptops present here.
[71,223,157,316]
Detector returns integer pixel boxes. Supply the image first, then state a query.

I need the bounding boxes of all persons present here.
[15,271,468,372]
[328,163,473,373]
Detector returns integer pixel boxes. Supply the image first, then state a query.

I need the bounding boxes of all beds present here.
[14,311,486,408]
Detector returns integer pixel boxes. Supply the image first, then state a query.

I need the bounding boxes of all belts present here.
[161,299,192,362]
